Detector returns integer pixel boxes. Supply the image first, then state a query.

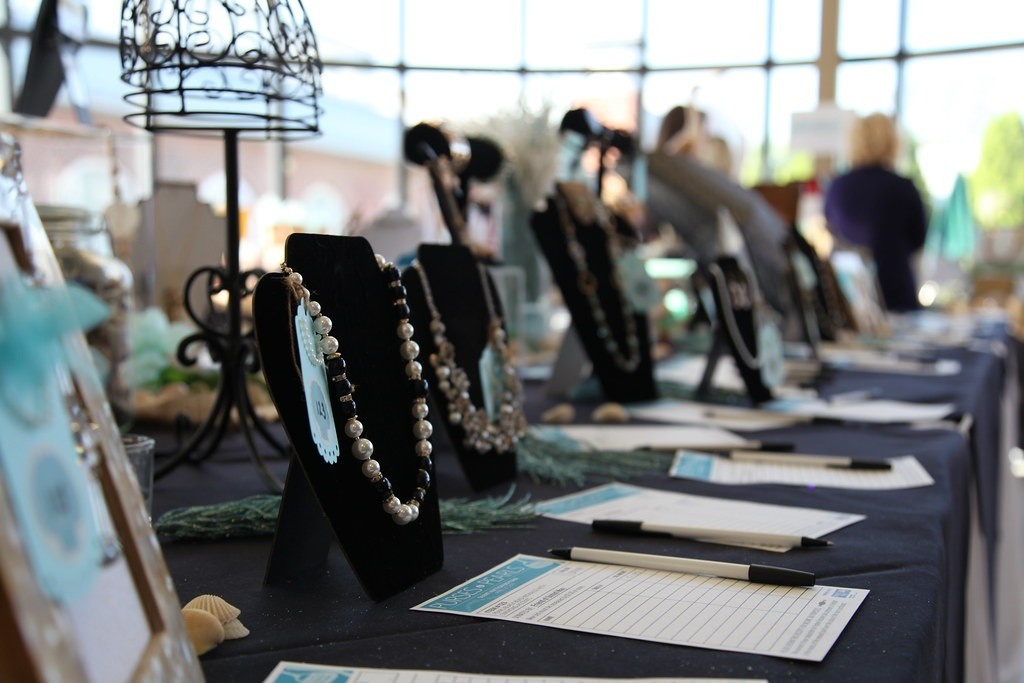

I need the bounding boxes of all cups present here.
[120,434,154,523]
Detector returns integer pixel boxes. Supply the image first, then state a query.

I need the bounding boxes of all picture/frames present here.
[0,177,206,683]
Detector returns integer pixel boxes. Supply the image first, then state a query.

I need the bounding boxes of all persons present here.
[827,114,926,313]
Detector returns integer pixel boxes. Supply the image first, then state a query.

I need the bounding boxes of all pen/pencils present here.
[719,450,892,474]
[548,546,817,587]
[592,519,836,549]
[634,442,796,454]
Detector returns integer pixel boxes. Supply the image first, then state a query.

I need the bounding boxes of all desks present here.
[0,316,1011,683]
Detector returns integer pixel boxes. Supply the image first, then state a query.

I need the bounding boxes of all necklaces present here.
[281,157,763,522]
